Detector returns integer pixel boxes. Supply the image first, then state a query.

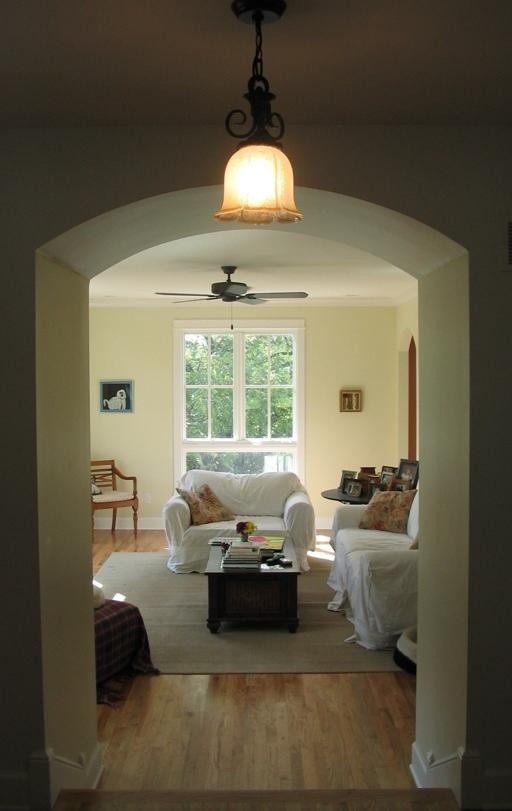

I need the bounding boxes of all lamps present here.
[213,1,311,225]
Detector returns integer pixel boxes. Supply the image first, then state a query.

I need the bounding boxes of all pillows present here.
[175,484,235,525]
[90,484,101,495]
[357,490,415,533]
[93,581,105,608]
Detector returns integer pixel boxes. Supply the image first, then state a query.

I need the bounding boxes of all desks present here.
[321,486,372,510]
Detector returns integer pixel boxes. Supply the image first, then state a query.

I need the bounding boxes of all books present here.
[221,540,263,568]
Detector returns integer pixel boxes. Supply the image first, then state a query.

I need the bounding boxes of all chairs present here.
[91,459,140,540]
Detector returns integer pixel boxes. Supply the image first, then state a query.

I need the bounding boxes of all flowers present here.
[236,522,257,535]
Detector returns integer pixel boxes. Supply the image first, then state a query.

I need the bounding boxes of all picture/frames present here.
[98,378,134,412]
[338,389,362,412]
[338,459,419,496]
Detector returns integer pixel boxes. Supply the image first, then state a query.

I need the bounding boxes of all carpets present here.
[93,552,408,674]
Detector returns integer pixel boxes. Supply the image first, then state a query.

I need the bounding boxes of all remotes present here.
[266,555,284,563]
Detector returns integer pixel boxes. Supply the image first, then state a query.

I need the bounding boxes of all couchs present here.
[91,599,158,706]
[329,489,420,647]
[162,469,316,574]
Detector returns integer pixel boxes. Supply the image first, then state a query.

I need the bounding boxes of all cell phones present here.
[279,558,292,566]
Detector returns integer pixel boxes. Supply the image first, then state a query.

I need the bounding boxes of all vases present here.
[240,533,248,542]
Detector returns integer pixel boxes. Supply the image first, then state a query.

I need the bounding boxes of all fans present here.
[155,265,308,309]
